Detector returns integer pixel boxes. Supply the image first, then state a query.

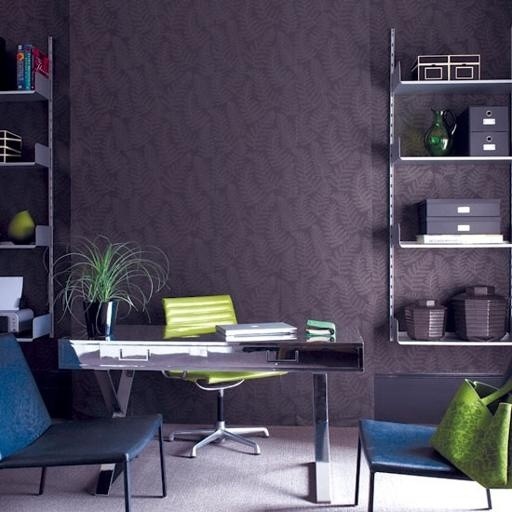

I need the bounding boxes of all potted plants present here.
[41,232,175,339]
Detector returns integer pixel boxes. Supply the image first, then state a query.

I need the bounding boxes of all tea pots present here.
[424,105,461,156]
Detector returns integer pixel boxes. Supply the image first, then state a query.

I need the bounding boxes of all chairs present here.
[353,357,512,512]
[0,334,168,512]
[161,292,292,459]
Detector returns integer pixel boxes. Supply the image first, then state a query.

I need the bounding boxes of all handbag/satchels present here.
[429,378,512,488]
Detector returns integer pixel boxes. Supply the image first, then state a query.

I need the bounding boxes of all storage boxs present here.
[455,105,510,156]
[411,55,481,80]
[417,198,501,234]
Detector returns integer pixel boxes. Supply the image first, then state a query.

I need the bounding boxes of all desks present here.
[59,321,364,504]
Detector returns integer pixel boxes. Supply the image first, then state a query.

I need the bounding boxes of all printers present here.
[0,288,35,338]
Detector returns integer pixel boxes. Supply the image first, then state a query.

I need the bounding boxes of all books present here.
[303,318,336,338]
[14,44,49,91]
[216,332,299,342]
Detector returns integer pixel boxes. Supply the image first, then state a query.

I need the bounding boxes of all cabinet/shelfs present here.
[390,26,511,347]
[0,34,71,346]
